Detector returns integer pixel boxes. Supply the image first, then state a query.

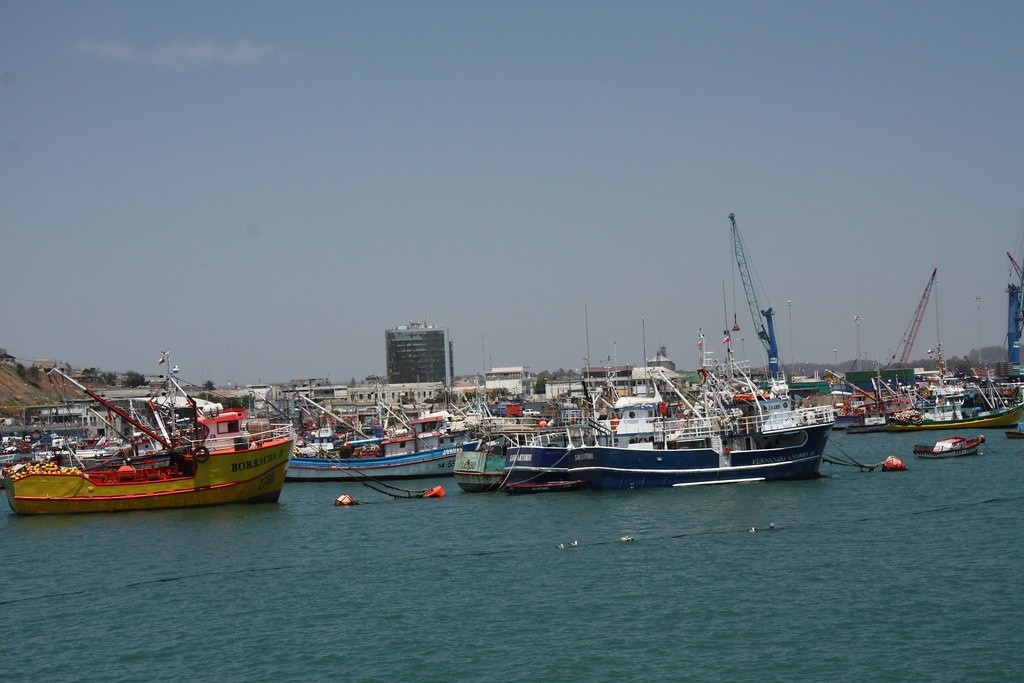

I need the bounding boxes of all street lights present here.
[852,314,864,374]
[785,300,796,374]
[973,296,984,380]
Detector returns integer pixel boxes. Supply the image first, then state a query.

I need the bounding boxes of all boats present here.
[911,433,987,459]
[1,309,1024,509]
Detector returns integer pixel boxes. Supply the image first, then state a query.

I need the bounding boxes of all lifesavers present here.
[638,437,648,443]
[374,450,382,457]
[192,446,210,463]
[362,451,367,456]
[368,450,374,456]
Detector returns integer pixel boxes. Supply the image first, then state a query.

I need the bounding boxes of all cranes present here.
[729,211,779,378]
[1005,251,1024,286]
[879,267,937,371]
